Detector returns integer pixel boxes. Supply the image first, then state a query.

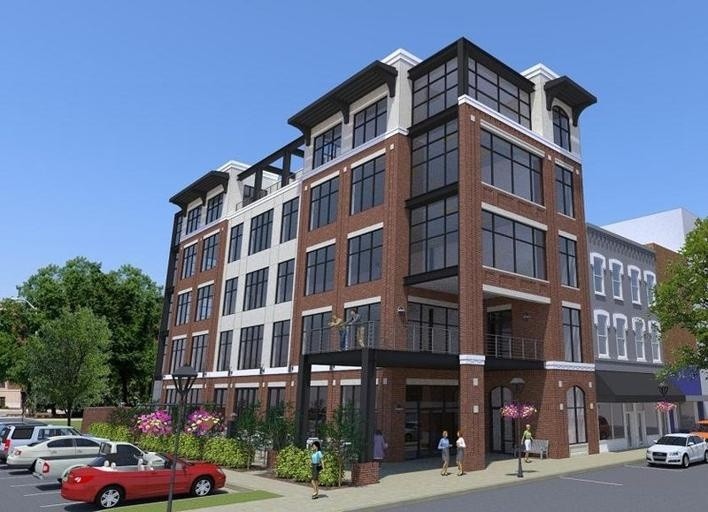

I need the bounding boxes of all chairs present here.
[103,458,155,472]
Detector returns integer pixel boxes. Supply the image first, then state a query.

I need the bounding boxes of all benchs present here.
[513,438,550,458]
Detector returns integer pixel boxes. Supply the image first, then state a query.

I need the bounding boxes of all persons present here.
[521,424,534,463]
[374,430,388,469]
[438,431,453,476]
[339,309,364,347]
[327,312,347,352]
[456,430,466,476]
[310,441,324,499]
